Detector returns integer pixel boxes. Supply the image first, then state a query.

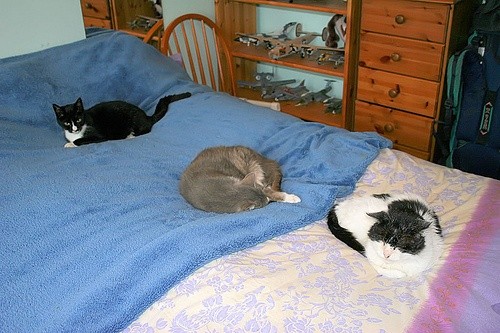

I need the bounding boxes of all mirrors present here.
[81,0,164,49]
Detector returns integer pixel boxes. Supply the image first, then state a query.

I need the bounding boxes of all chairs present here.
[160,13,236,96]
[143,21,162,48]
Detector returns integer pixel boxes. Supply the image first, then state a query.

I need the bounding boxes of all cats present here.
[327,192,444,279]
[52,92,192,148]
[178,145,302,215]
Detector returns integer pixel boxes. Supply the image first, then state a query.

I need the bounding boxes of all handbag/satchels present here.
[467,1,500,59]
[444,47,499,149]
[434,132,500,179]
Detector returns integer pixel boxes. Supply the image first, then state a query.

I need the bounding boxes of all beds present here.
[0,30,500,333]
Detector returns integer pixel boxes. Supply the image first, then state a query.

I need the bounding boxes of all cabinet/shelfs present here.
[350,0,452,162]
[111,0,165,48]
[211,1,359,131]
[80,0,114,32]
